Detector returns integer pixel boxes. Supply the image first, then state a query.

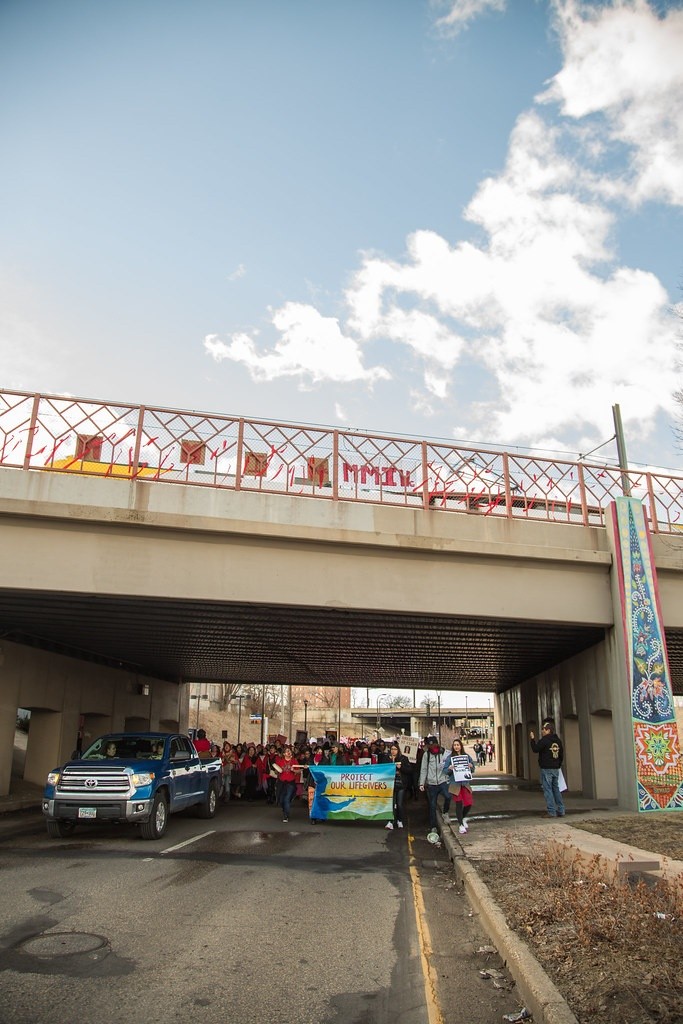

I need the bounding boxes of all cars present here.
[470,728,481,736]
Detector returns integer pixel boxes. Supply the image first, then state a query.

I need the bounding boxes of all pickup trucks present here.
[42,732,223,840]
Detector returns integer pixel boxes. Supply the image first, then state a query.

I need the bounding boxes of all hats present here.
[540,722,553,729]
[427,737,438,746]
[310,738,317,745]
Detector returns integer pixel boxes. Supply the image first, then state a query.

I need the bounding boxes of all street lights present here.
[143,681,153,732]
[488,699,490,712]
[466,696,468,718]
[229,694,251,744]
[304,699,308,731]
[191,694,208,730]
[376,693,387,740]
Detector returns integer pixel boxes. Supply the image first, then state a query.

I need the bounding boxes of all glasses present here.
[391,748,398,750]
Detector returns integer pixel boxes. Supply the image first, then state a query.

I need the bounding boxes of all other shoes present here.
[542,813,563,818]
[312,820,316,825]
[248,799,253,802]
[283,819,288,823]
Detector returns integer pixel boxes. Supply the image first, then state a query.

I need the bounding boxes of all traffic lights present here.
[490,716,494,727]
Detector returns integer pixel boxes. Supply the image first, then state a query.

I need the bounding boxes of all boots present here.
[225,792,230,803]
[266,786,275,805]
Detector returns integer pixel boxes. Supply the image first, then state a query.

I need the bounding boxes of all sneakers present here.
[462,818,468,829]
[458,824,467,834]
[431,827,437,833]
[397,820,403,828]
[385,821,394,830]
[441,813,451,824]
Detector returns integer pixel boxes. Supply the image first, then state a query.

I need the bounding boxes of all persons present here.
[530,723,566,818]
[192,728,452,832]
[443,739,475,833]
[473,740,495,766]
[143,740,165,759]
[96,743,119,759]
